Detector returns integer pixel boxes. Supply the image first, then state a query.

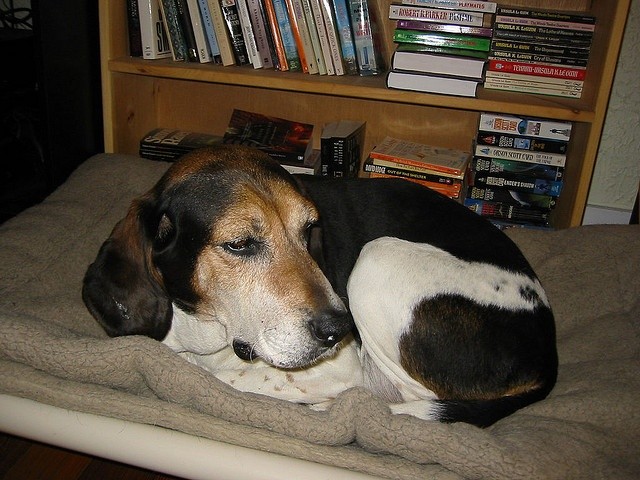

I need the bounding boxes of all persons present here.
[126,1,391,78]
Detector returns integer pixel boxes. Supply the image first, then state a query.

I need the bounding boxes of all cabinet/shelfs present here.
[99,0,633,227]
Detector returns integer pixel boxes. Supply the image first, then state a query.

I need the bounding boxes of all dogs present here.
[82,145,557,428]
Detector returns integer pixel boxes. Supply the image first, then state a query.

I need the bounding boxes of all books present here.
[359,136,471,205]
[386,1,598,101]
[462,111,574,230]
[139,128,224,161]
[223,109,321,175]
[320,119,367,178]
